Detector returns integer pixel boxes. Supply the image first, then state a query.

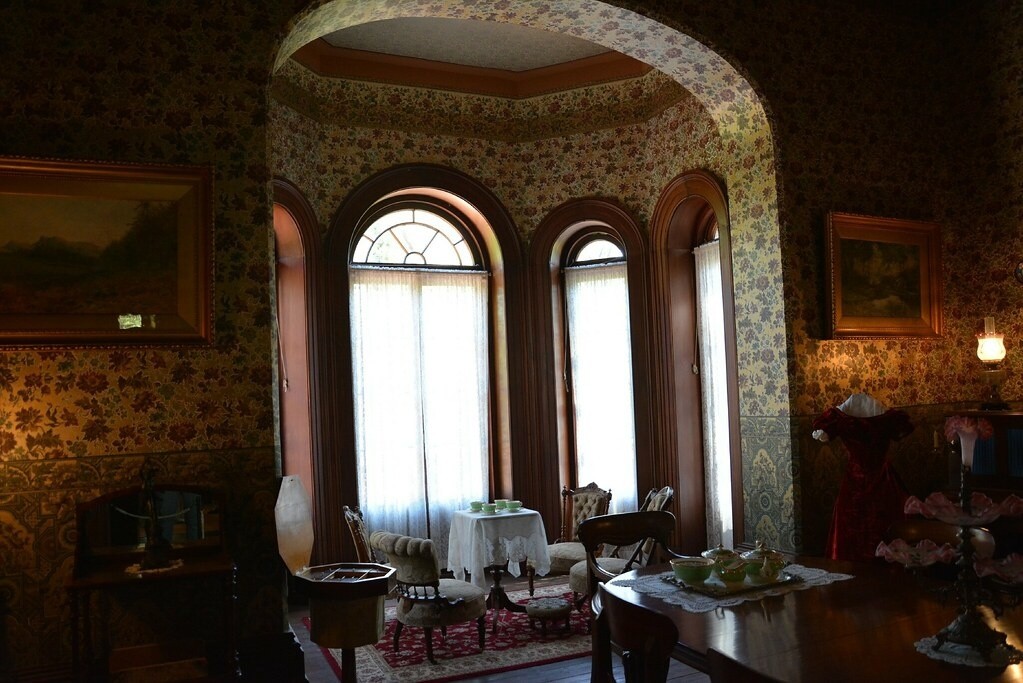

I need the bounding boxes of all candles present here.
[933,430,940,449]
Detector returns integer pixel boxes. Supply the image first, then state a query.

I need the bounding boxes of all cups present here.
[718,558,747,585]
[506,500,522,511]
[702,543,738,574]
[494,499,506,509]
[471,502,486,512]
[481,504,496,513]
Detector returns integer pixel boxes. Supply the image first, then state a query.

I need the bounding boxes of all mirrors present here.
[70,480,227,576]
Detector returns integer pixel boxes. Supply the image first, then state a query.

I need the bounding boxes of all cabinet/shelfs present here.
[947,409,1023,535]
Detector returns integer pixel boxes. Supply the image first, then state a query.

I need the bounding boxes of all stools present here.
[527,597,572,638]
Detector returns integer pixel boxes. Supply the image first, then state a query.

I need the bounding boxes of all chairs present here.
[526,482,675,635]
[343,505,488,665]
[577,509,708,683]
[885,519,997,582]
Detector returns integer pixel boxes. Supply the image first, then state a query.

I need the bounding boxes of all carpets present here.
[305,580,597,683]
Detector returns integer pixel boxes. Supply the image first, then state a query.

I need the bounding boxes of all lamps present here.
[974,316,1014,411]
[129,458,185,576]
[871,413,1023,669]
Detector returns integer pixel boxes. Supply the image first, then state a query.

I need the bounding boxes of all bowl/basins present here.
[670,558,715,585]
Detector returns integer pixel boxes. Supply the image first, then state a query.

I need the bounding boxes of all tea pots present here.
[741,541,786,583]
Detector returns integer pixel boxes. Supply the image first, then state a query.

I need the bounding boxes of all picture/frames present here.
[0,150,218,355]
[825,209,950,341]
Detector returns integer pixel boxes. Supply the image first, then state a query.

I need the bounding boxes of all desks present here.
[65,549,243,683]
[447,500,551,635]
[590,555,1023,683]
